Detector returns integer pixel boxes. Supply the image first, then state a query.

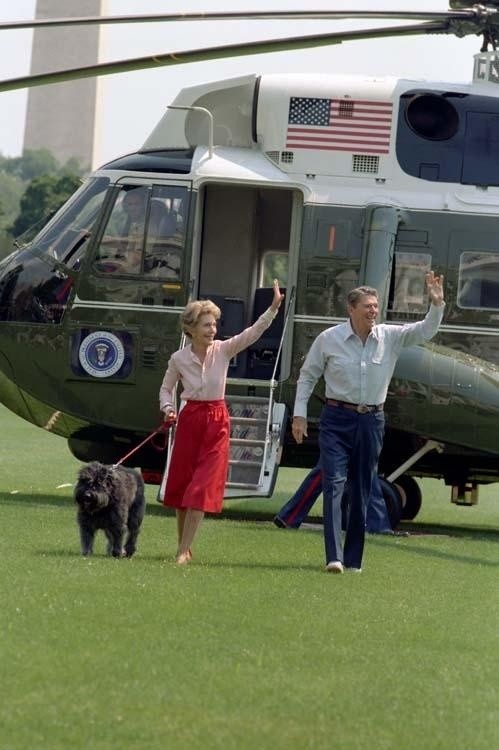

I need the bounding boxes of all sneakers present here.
[326,560,362,575]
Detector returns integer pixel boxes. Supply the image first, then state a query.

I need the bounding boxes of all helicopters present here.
[0,0,499,530]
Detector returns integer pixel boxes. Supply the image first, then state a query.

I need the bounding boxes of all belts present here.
[326,399,384,414]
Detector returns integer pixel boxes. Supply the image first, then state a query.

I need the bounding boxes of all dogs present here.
[74,461,145,559]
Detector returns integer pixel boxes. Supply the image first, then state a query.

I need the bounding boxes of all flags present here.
[285,94,394,155]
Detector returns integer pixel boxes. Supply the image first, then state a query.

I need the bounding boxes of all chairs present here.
[196,284,287,378]
[150,200,177,253]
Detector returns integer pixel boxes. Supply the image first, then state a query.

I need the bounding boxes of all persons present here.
[149,199,169,228]
[79,190,166,280]
[272,445,411,538]
[292,268,446,575]
[157,278,286,568]
